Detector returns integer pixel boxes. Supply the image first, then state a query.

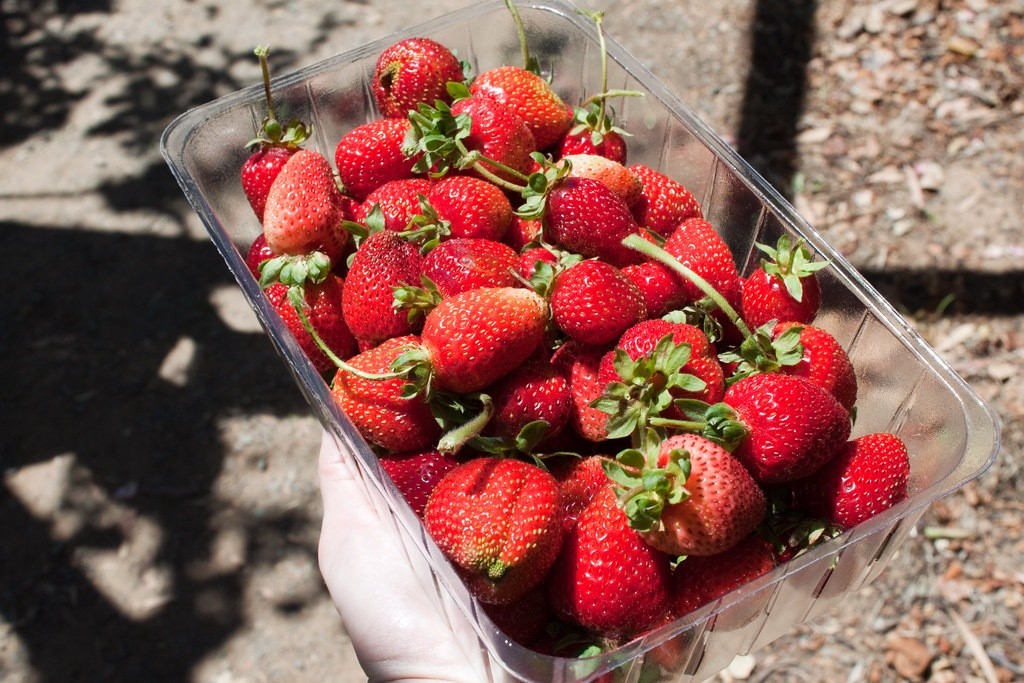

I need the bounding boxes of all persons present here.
[313,429,528,681]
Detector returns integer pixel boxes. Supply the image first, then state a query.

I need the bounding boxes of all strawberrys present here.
[242,0,910,683]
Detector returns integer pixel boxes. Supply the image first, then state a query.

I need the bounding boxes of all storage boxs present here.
[161,1,996,683]
